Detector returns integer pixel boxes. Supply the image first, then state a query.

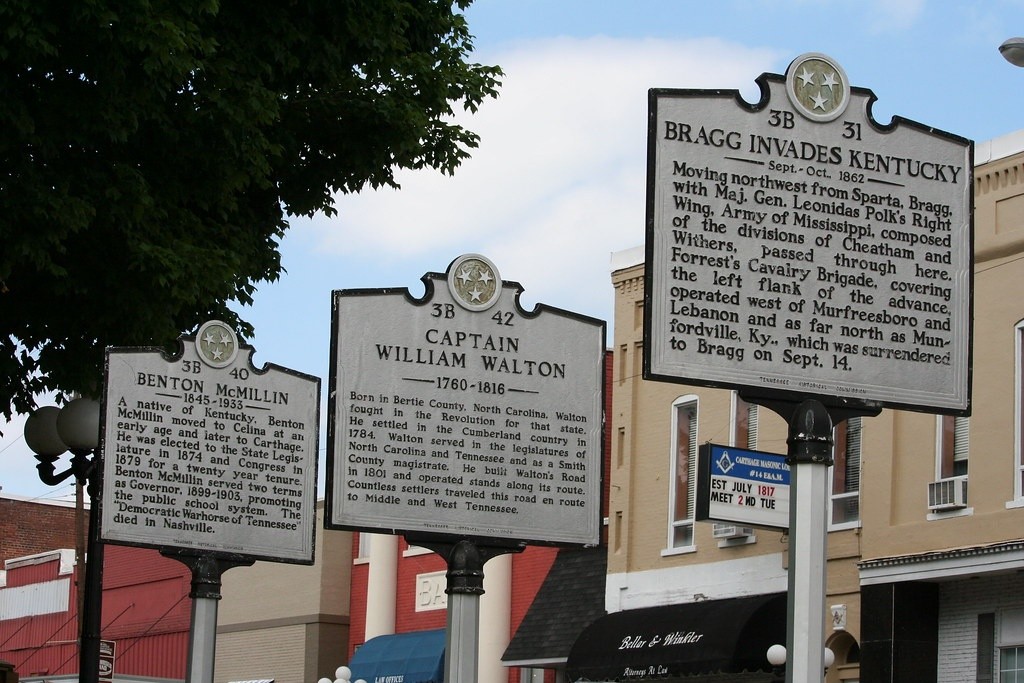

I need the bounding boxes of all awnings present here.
[564,590,787,683]
[348,629,446,683]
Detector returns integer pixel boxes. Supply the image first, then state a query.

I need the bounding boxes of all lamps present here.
[712,523,753,539]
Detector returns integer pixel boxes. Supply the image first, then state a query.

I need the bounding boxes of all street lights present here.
[23,397,104,683]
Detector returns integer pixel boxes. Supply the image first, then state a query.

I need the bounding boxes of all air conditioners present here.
[928,478,968,510]
